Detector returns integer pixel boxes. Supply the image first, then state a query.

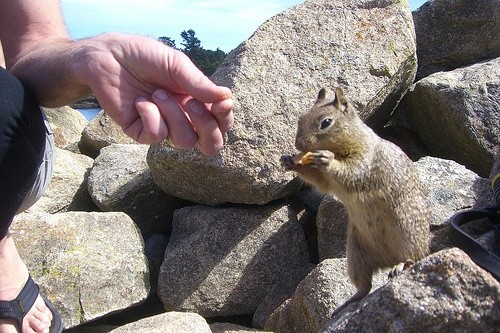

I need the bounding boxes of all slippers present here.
[0,273,63,333]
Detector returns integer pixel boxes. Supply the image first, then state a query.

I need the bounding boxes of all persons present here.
[0,0,234,333]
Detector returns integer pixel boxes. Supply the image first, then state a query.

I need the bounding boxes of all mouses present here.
[278,87,432,319]
